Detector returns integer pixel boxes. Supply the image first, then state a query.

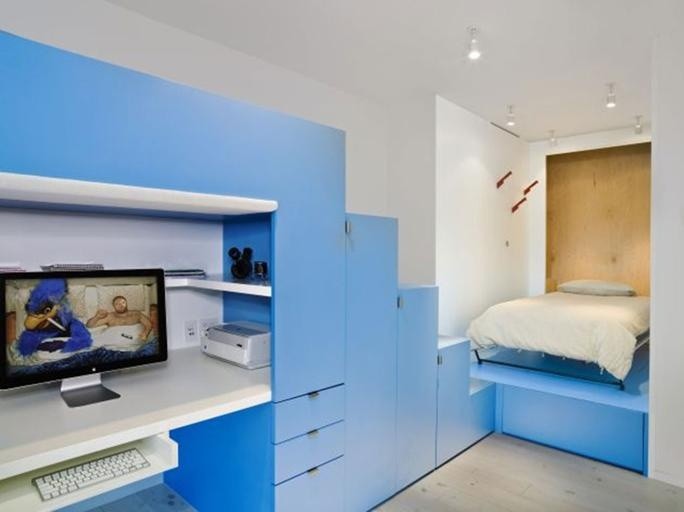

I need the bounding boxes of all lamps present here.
[459,22,648,144]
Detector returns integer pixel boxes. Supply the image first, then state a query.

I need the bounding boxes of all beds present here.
[466,274,650,391]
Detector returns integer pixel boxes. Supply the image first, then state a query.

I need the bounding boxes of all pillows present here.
[549,277,638,298]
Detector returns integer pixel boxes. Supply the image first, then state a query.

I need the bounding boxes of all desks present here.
[1,342,279,511]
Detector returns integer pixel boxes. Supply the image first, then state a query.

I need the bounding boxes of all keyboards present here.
[28,445,154,506]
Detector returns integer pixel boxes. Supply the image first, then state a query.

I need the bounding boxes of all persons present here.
[17,279,161,360]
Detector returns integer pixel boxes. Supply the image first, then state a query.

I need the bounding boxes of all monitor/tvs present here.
[0,268,168,408]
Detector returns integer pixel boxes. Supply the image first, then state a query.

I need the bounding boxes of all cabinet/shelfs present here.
[435,332,497,470]
[218,109,348,406]
[340,207,402,511]
[161,377,345,510]
[394,281,441,497]
